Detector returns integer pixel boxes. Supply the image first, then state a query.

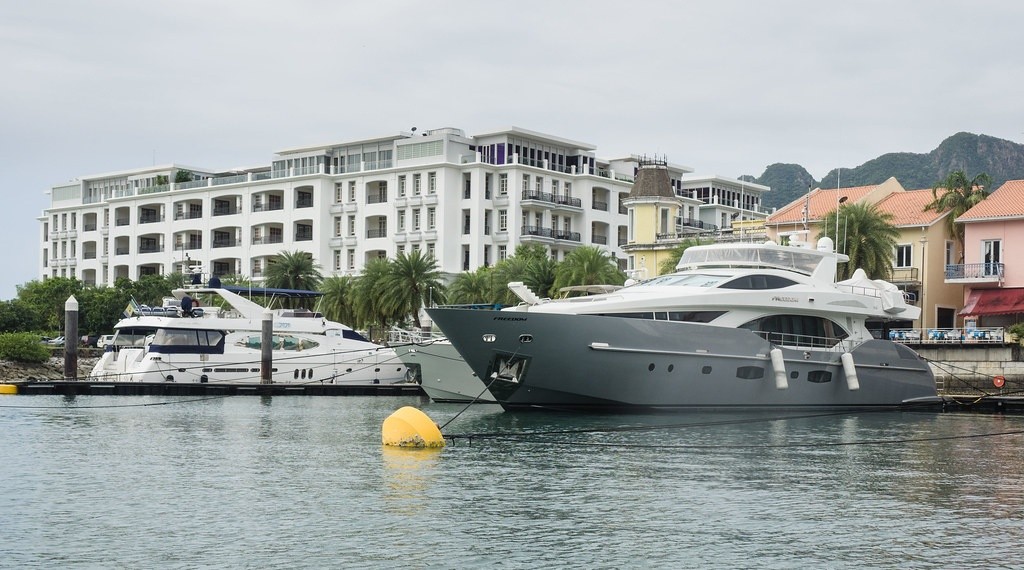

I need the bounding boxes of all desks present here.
[890,331,917,332]
[932,330,950,332]
[974,330,1001,332]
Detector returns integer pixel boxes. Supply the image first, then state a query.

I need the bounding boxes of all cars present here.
[48,336,65,346]
[134,333,156,346]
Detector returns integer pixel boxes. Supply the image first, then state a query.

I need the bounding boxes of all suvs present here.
[97,334,132,350]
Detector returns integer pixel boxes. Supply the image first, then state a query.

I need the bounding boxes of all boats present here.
[386,325,501,405]
[90,243,421,385]
[421,157,971,415]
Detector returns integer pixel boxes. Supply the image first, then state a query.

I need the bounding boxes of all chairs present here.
[888,328,1001,344]
